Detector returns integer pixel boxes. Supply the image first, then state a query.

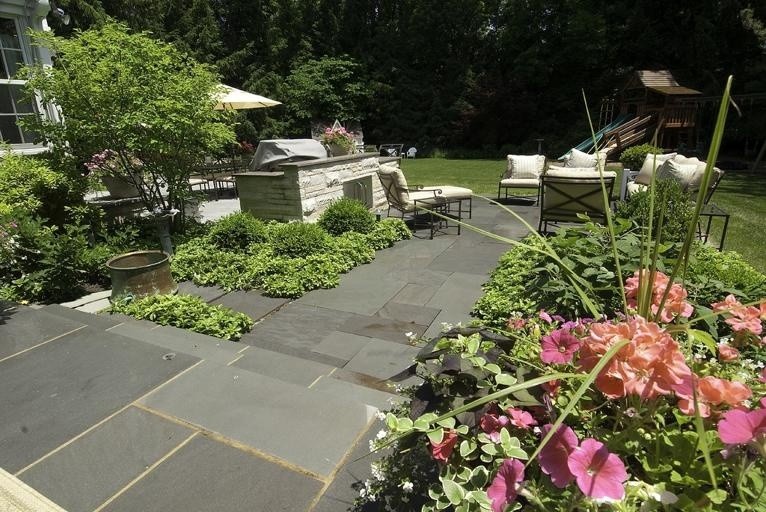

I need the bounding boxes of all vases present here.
[101,174,143,198]
[330,144,348,157]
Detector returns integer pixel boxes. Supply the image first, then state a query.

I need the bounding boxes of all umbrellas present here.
[196,81,282,173]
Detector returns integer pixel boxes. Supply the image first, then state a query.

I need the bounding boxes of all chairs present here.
[497,150,726,234]
[375,164,473,227]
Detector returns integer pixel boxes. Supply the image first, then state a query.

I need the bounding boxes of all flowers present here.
[322,127,356,154]
[83,150,143,176]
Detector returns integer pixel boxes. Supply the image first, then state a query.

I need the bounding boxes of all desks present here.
[698,204,730,252]
[413,197,461,239]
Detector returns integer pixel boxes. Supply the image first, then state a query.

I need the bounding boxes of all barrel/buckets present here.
[101,170,145,199]
